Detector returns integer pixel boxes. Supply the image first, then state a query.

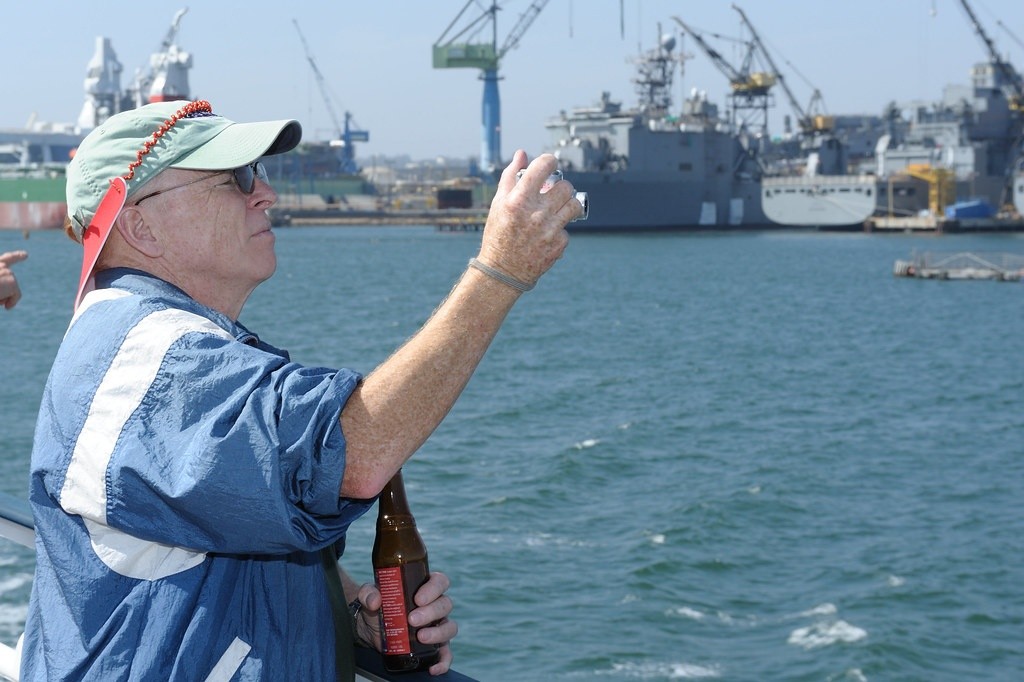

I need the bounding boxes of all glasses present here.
[134,160,272,205]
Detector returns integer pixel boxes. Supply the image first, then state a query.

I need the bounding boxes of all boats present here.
[895,248,1023,281]
[493,21,876,229]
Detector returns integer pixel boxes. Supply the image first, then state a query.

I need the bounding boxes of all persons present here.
[20,100,583,682]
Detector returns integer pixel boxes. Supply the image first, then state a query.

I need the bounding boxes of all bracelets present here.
[348,598,370,649]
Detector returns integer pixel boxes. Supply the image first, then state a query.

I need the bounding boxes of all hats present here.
[64,100,303,247]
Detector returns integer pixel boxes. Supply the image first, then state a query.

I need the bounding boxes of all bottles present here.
[372,467,440,673]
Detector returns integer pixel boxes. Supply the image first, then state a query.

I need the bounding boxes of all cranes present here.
[431,0,551,181]
[959,0,1024,111]
[292,18,368,173]
[670,3,834,133]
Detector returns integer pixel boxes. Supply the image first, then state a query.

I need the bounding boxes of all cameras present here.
[516,168,590,222]
